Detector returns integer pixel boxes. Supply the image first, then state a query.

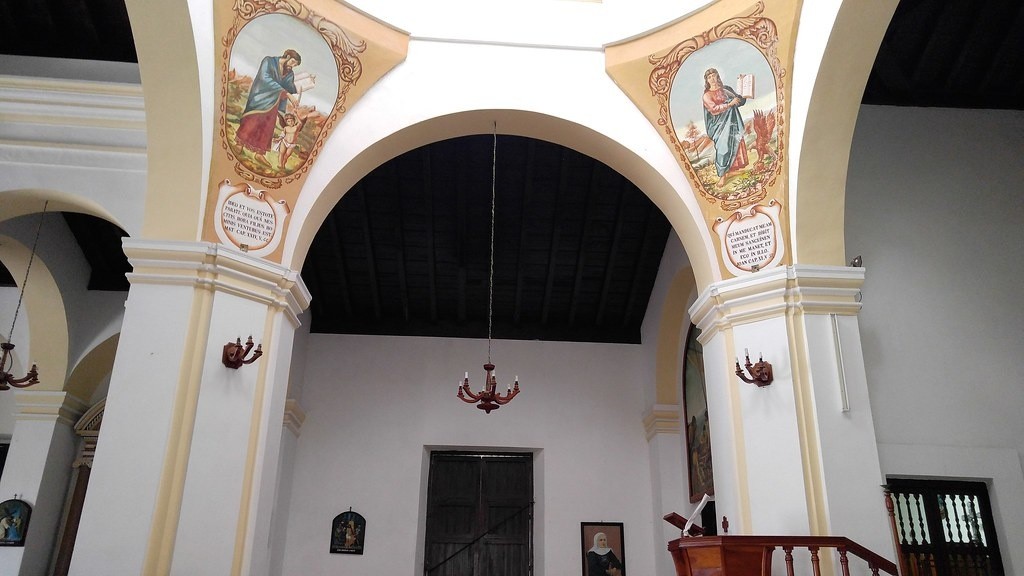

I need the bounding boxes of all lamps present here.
[222,335,263,369]
[457,121,521,415]
[735,348,773,387]
[0,200,48,391]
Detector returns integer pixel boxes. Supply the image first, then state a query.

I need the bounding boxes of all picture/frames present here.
[581,522,626,576]
